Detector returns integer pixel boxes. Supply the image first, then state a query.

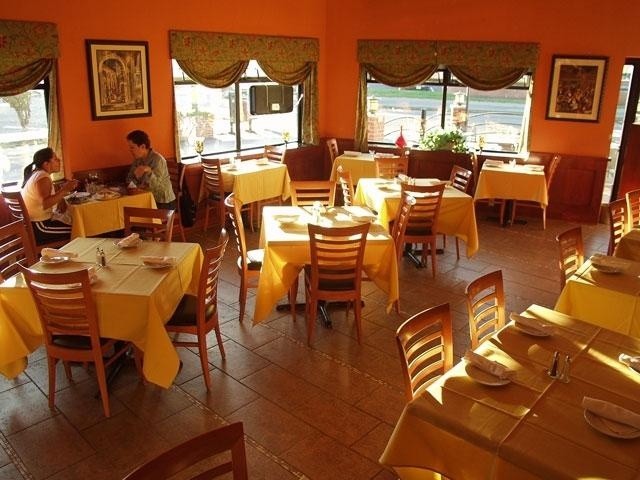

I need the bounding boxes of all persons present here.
[124,129,178,215]
[18,146,81,243]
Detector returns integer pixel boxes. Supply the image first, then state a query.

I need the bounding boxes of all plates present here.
[68,276,98,288]
[40,254,68,263]
[70,191,120,201]
[583,408,640,440]
[593,263,620,274]
[464,363,511,388]
[274,215,297,225]
[144,262,170,269]
[344,151,393,159]
[114,238,143,248]
[515,321,551,337]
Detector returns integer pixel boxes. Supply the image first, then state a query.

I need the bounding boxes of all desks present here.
[5,237,203,409]
[330,152,402,186]
[255,204,400,331]
[358,175,481,261]
[476,160,549,220]
[64,181,155,240]
[216,161,292,230]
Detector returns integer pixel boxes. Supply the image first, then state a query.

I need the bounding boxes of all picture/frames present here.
[84,37,152,120]
[544,54,610,123]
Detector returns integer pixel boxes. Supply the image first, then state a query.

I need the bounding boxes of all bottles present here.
[408,177,417,187]
[548,349,573,385]
[94,248,108,268]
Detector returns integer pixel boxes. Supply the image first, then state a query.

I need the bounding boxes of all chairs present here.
[1,220,36,281]
[625,188,640,232]
[511,155,560,230]
[463,268,507,347]
[468,152,505,226]
[306,223,371,347]
[198,157,253,233]
[289,181,337,210]
[263,145,286,165]
[225,192,298,325]
[336,166,355,207]
[139,234,229,394]
[122,205,176,244]
[556,227,591,291]
[394,304,454,403]
[393,183,447,280]
[327,137,340,161]
[443,164,473,263]
[17,260,145,417]
[156,161,186,241]
[2,191,70,257]
[603,197,631,259]
[124,420,250,480]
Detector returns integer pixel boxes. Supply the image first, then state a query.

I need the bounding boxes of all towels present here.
[617,351,640,372]
[459,347,517,381]
[579,394,639,432]
[509,311,560,336]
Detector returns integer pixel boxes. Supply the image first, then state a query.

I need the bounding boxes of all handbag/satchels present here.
[177,163,196,227]
